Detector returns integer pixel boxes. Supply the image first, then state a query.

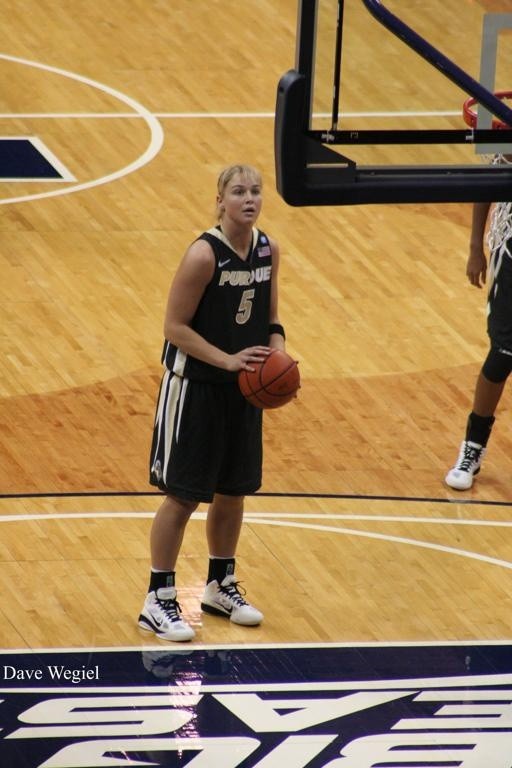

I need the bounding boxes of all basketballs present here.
[239,349,300,408]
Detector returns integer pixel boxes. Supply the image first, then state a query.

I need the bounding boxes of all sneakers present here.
[138,587,196,642]
[201,574,263,626]
[445,440,484,491]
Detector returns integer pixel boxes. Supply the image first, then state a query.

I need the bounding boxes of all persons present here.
[442,153,512,493]
[135,160,302,641]
